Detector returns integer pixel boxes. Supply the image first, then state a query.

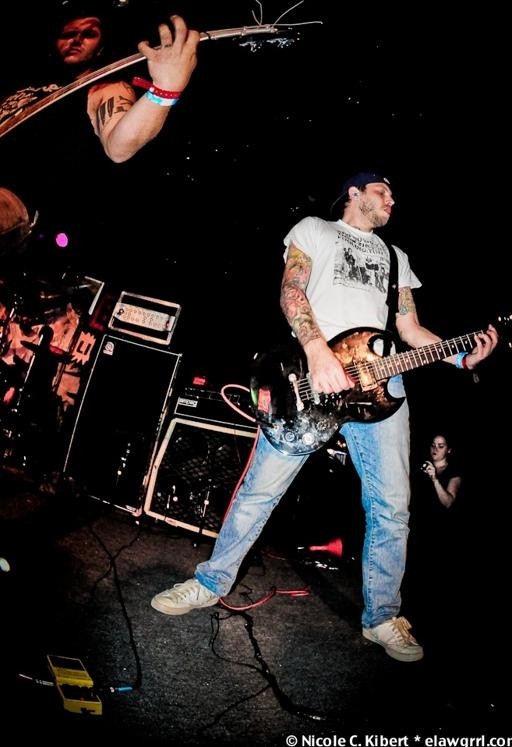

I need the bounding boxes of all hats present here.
[330,169,393,216]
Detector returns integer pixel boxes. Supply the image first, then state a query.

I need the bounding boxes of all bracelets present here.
[460,353,472,372]
[146,89,180,108]
[131,76,183,97]
[455,350,468,371]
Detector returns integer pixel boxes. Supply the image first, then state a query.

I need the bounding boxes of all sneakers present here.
[360,616,424,663]
[151,578,218,614]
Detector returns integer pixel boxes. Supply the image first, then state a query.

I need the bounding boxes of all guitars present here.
[0,24,276,142]
[249,314,512,455]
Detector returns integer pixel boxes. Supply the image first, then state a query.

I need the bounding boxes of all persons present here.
[0,2,206,283]
[150,168,503,664]
[412,425,463,559]
